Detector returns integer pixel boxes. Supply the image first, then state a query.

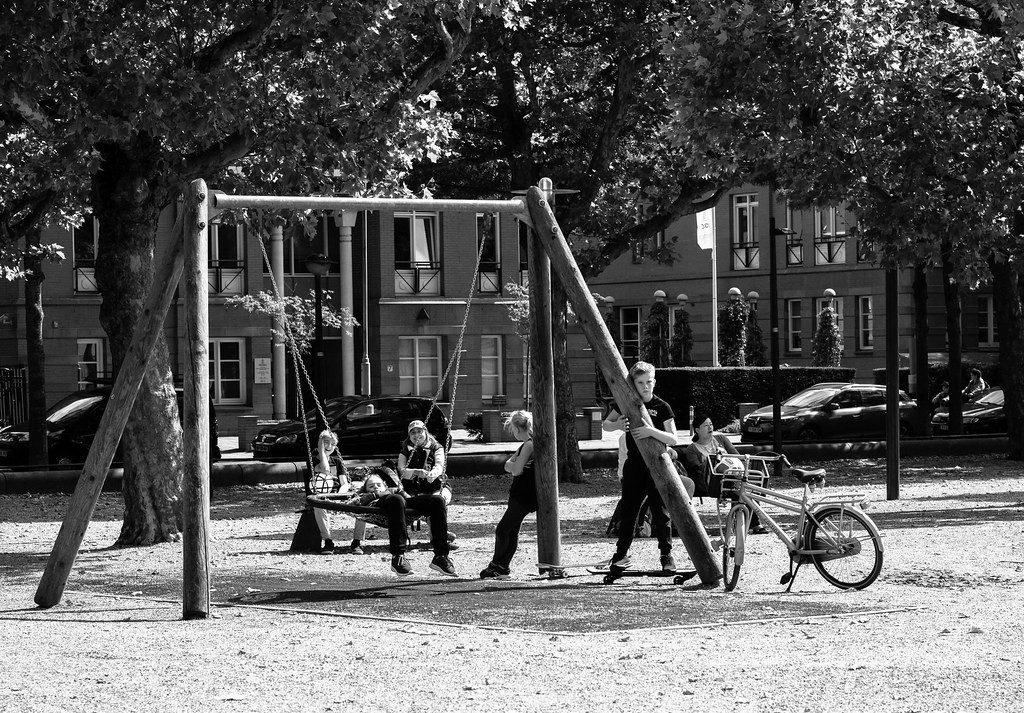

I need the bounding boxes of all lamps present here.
[415,308,431,322]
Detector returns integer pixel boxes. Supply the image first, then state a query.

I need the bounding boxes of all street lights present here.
[653,289,689,368]
[297,252,337,452]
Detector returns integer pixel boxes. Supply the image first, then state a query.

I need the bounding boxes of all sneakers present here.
[429,556,458,576]
[660,556,677,572]
[609,553,631,570]
[391,555,414,575]
[480,561,510,580]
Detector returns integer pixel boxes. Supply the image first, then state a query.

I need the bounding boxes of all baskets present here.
[703,454,770,498]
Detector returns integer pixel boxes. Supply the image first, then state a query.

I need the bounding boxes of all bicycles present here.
[707,444,884,593]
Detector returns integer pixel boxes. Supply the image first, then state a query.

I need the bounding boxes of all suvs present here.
[3,371,222,464]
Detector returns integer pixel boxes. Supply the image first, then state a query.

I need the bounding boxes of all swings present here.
[240,208,492,530]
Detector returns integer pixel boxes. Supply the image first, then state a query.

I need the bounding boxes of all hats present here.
[408,420,423,432]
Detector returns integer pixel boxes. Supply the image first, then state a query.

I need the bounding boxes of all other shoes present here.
[351,542,363,554]
[447,532,456,541]
[321,542,335,554]
[448,542,459,549]
[753,528,768,533]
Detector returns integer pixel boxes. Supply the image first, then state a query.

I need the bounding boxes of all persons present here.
[306,420,457,578]
[602,362,768,574]
[479,411,539,580]
[932,368,990,413]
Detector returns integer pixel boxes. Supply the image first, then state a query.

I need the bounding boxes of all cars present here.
[250,391,453,459]
[740,381,935,442]
[930,386,1007,435]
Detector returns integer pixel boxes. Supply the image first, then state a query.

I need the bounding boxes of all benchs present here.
[300,465,421,533]
